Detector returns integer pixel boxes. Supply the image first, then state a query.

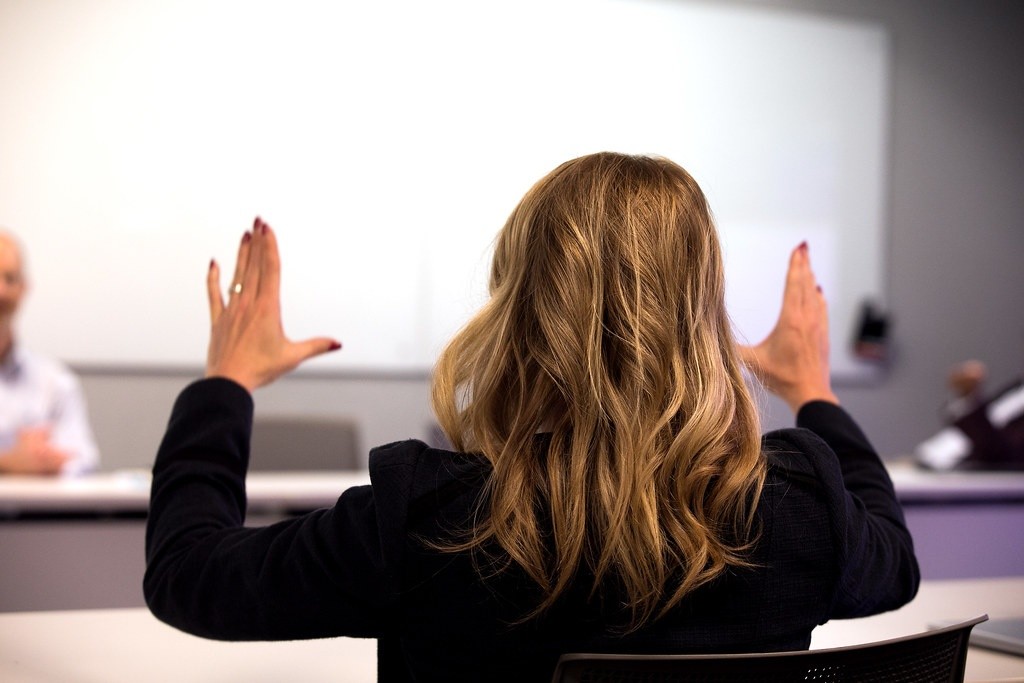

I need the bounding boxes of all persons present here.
[0,226,102,482]
[942,354,1024,471]
[140,152,921,683]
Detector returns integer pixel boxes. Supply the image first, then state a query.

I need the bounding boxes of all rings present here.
[229,281,242,294]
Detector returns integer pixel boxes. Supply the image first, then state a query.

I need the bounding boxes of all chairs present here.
[557,615,994,683]
[249,420,363,467]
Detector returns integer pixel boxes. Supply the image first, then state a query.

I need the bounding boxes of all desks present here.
[0,575,1023,682]
[1,467,1024,613]
